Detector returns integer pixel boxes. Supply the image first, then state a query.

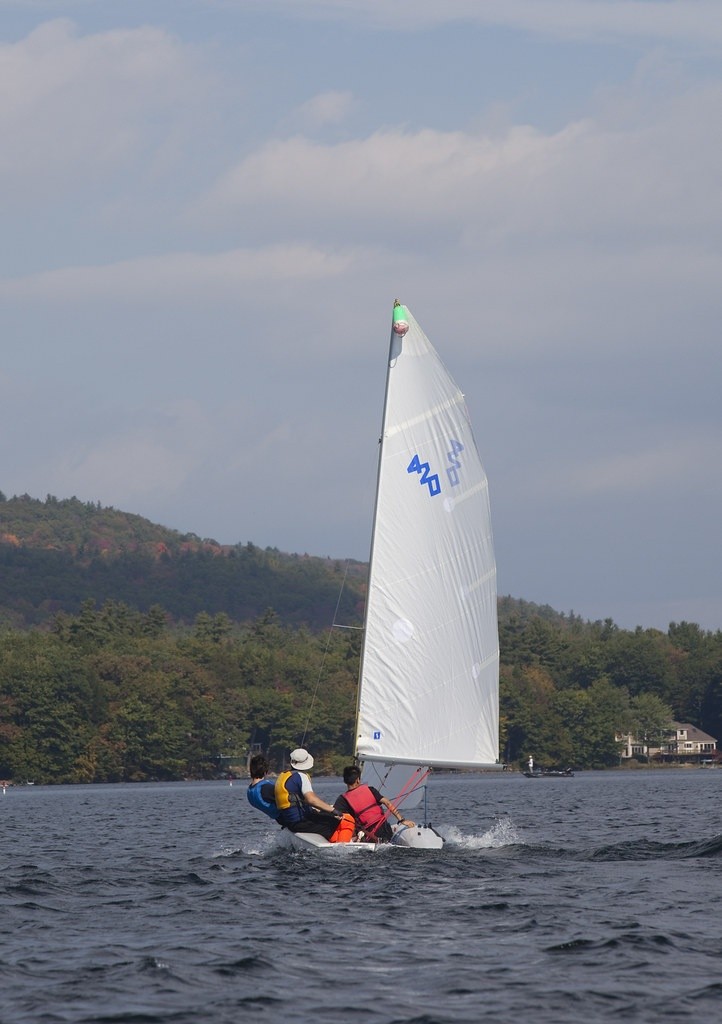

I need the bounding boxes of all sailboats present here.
[285,296,504,854]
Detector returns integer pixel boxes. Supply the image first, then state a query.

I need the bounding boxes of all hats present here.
[529,755,532,758]
[289,749,314,770]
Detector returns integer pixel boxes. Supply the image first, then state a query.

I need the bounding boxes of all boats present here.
[522,769,575,779]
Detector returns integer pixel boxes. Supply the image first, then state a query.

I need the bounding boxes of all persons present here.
[275,749,358,843]
[527,755,534,773]
[247,755,287,828]
[334,766,415,843]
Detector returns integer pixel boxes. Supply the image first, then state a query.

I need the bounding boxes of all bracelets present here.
[397,818,405,825]
